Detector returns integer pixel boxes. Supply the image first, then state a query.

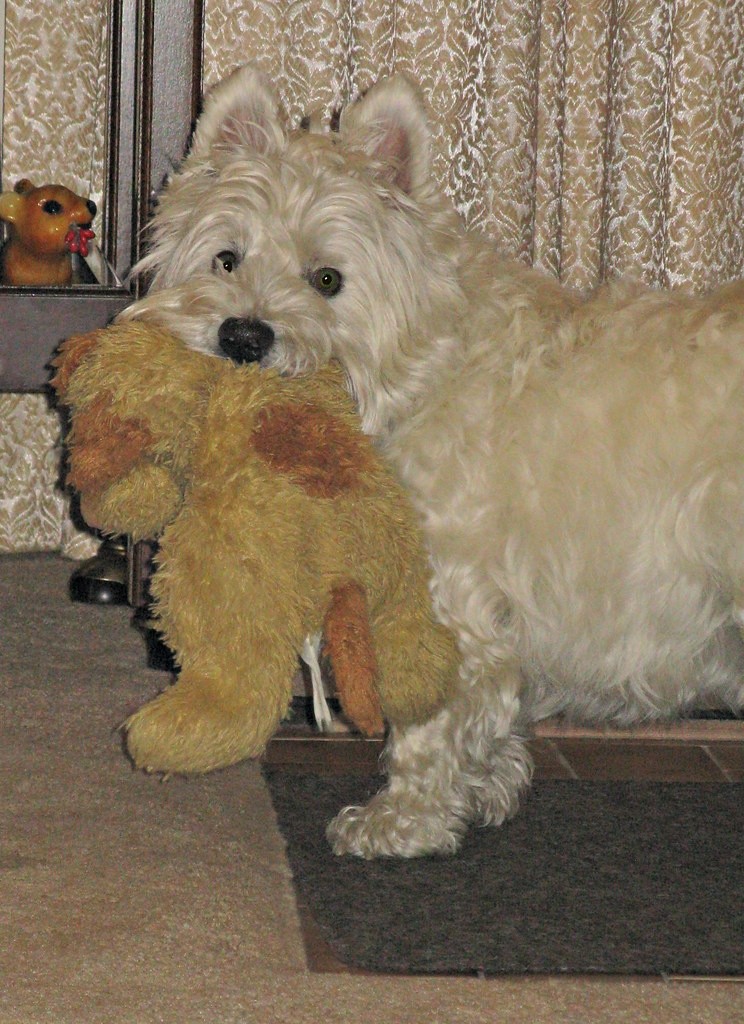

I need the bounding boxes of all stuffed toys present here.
[43,313,462,774]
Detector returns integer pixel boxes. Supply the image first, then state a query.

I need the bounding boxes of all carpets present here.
[265,771,743,975]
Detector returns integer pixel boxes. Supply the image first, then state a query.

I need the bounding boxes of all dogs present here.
[111,61,744,862]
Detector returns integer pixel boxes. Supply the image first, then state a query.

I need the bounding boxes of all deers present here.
[0,178,97,287]
